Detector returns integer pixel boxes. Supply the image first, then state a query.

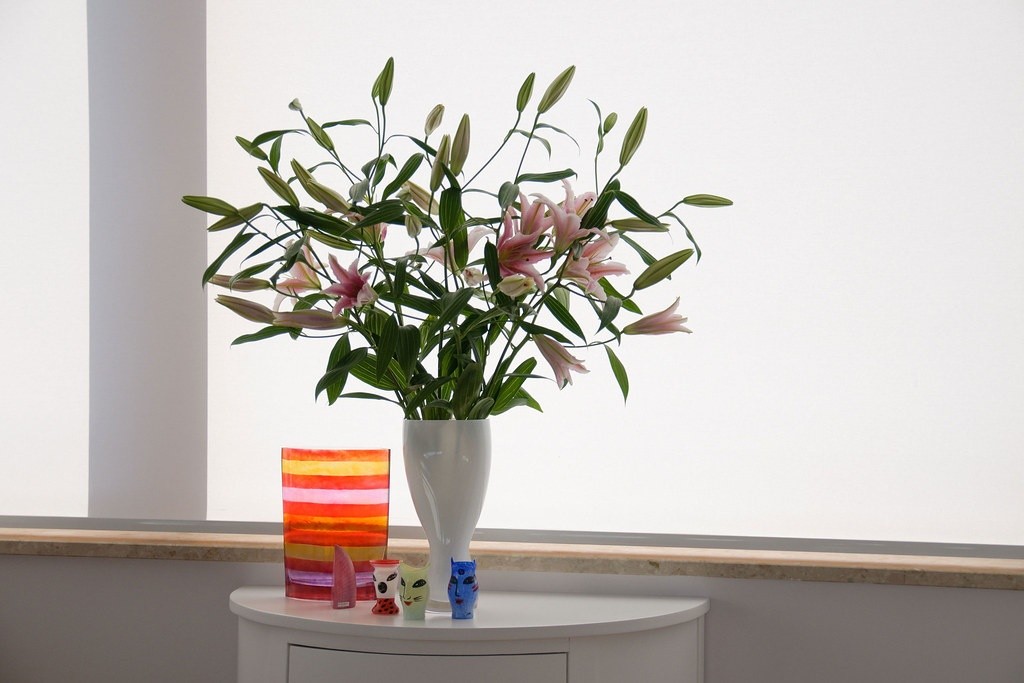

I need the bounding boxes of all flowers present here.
[181,57,734,420]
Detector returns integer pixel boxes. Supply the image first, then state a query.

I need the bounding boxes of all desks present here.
[229,585,710,683]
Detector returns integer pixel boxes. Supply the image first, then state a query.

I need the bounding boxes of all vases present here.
[403,419,491,603]
